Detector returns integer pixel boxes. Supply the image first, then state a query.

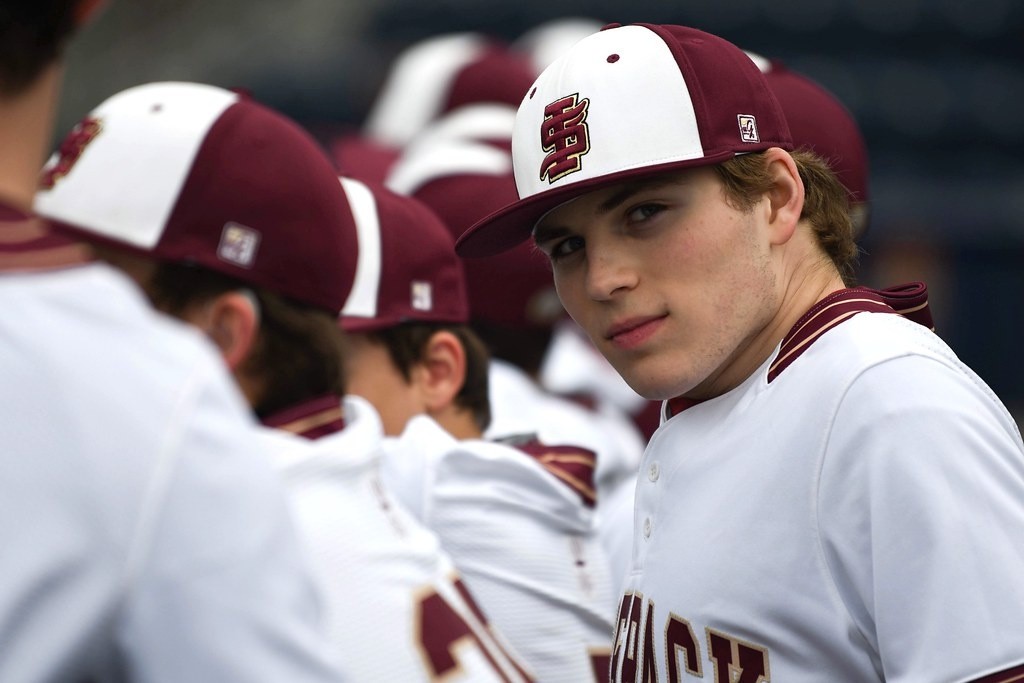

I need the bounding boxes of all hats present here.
[333,17,595,322]
[337,173,470,332]
[34,79,357,314]
[763,60,868,204]
[455,20,796,262]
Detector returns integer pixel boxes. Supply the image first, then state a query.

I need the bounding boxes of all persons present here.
[453,23,1024,683]
[0,0,868,683]
